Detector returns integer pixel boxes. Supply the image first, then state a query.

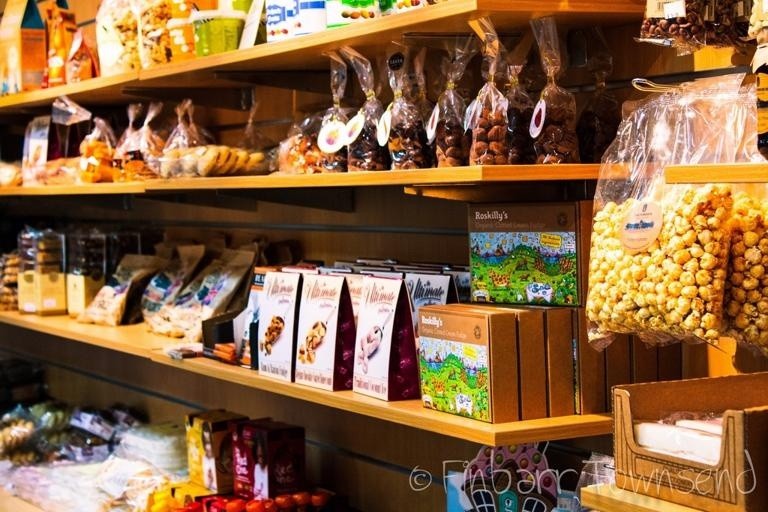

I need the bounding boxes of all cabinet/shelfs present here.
[0,0,767,512]
